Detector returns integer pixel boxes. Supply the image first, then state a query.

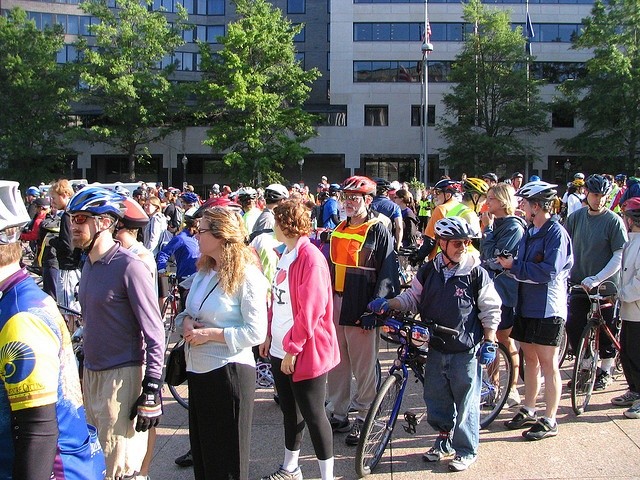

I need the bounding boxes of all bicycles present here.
[158,270,193,352]
[18,229,42,285]
[568,281,623,415]
[355,306,513,478]
[166,338,381,412]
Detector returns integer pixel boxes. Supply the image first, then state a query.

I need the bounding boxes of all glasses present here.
[340,192,365,201]
[198,229,214,232]
[394,197,398,198]
[446,239,472,248]
[434,191,444,195]
[72,214,98,224]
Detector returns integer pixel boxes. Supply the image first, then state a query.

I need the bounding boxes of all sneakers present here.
[176,450,192,465]
[484,388,502,407]
[522,418,558,440]
[611,391,639,405]
[126,474,149,480]
[345,419,365,446]
[507,388,520,408]
[327,413,350,431]
[623,395,640,417]
[423,437,455,462]
[449,453,477,471]
[261,464,303,480]
[504,408,537,429]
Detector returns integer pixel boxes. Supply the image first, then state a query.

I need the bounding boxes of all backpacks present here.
[317,199,332,243]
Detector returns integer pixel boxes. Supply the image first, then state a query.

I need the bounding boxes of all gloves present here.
[581,277,599,291]
[593,369,611,391]
[130,377,163,432]
[368,298,390,316]
[475,339,498,365]
[356,308,377,330]
[568,368,590,387]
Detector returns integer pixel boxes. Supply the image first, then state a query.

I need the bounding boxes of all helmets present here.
[239,186,257,199]
[1,180,32,244]
[617,174,625,180]
[463,178,489,197]
[573,179,584,186]
[116,196,149,228]
[575,173,584,178]
[530,176,540,181]
[620,197,640,221]
[34,199,49,207]
[514,180,558,202]
[66,185,126,219]
[185,207,198,217]
[434,217,477,239]
[511,172,523,180]
[25,186,40,196]
[329,184,342,190]
[182,192,197,204]
[338,176,377,195]
[373,178,395,191]
[193,197,245,218]
[482,173,498,181]
[264,183,289,200]
[433,179,458,192]
[584,173,612,195]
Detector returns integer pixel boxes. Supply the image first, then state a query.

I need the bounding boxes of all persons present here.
[1,177,108,479]
[175,205,268,480]
[258,202,344,480]
[158,216,201,306]
[22,198,47,241]
[25,186,41,203]
[368,217,502,471]
[495,181,575,441]
[66,186,165,479]
[600,196,640,418]
[50,179,82,329]
[41,201,58,301]
[326,176,397,447]
[478,182,520,409]
[562,174,628,388]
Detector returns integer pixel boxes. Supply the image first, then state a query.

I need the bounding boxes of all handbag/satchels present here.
[164,343,187,386]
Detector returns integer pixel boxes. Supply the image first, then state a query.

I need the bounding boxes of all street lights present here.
[297,156,305,187]
[181,155,188,193]
[421,42,433,185]
